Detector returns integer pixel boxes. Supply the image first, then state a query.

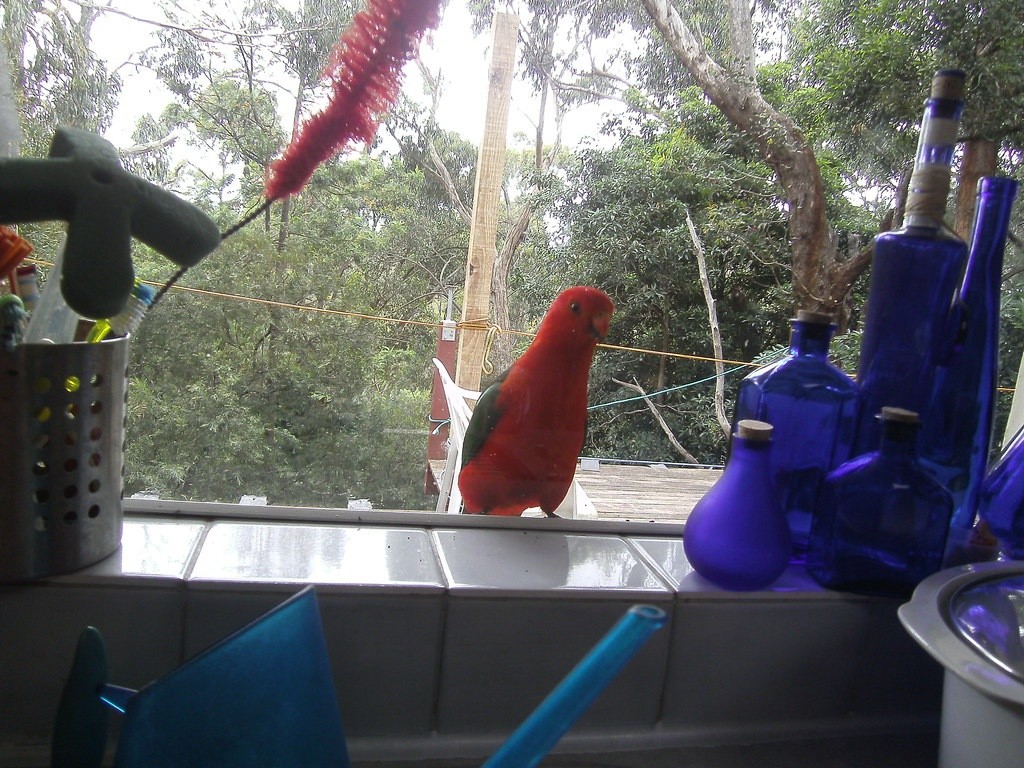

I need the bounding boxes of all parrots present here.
[458,285,615,520]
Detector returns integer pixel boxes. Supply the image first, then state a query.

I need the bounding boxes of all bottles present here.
[852,70,968,456]
[727,310,862,552]
[808,407,953,597]
[683,419,793,590]
[914,177,1017,569]
[979,427,1024,560]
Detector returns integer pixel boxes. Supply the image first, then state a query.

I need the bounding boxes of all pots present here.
[897,561,1024,768]
